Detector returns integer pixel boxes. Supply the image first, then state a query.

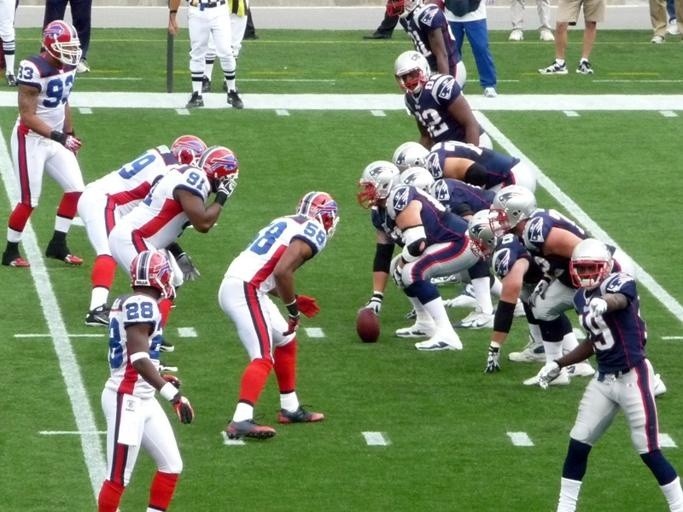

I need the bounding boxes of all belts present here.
[197,0,225,9]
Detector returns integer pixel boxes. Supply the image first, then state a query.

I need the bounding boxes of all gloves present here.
[590,297,607,317]
[526,274,551,306]
[536,360,561,390]
[280,294,320,336]
[214,176,237,198]
[392,265,403,289]
[175,251,200,281]
[483,340,500,373]
[364,291,384,314]
[63,134,81,152]
[160,375,194,425]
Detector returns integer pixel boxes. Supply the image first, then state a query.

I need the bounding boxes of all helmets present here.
[40,20,82,66]
[129,250,176,300]
[357,159,400,209]
[467,209,497,261]
[296,191,339,240]
[390,141,435,194]
[488,184,537,240]
[197,145,238,193]
[568,238,614,290]
[169,135,207,167]
[393,51,431,96]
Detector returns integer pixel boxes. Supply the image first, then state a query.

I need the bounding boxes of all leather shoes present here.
[363,30,391,39]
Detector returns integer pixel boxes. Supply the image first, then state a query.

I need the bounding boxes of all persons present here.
[218,190,343,439]
[76,133,239,354]
[1,20,89,268]
[96,250,192,511]
[0,0,251,110]
[351,0,682,399]
[522,237,679,512]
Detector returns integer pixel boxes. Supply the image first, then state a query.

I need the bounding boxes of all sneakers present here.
[1,252,29,267]
[414,335,463,351]
[507,343,666,399]
[201,75,239,93]
[432,274,526,330]
[276,404,325,423]
[6,59,89,87]
[484,86,497,98]
[227,89,243,108]
[395,324,433,338]
[650,17,678,44]
[509,25,592,75]
[226,419,276,439]
[45,239,82,265]
[158,340,178,372]
[83,303,110,327]
[185,89,203,109]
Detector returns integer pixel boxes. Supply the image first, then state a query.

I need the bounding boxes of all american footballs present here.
[356,310,381,344]
